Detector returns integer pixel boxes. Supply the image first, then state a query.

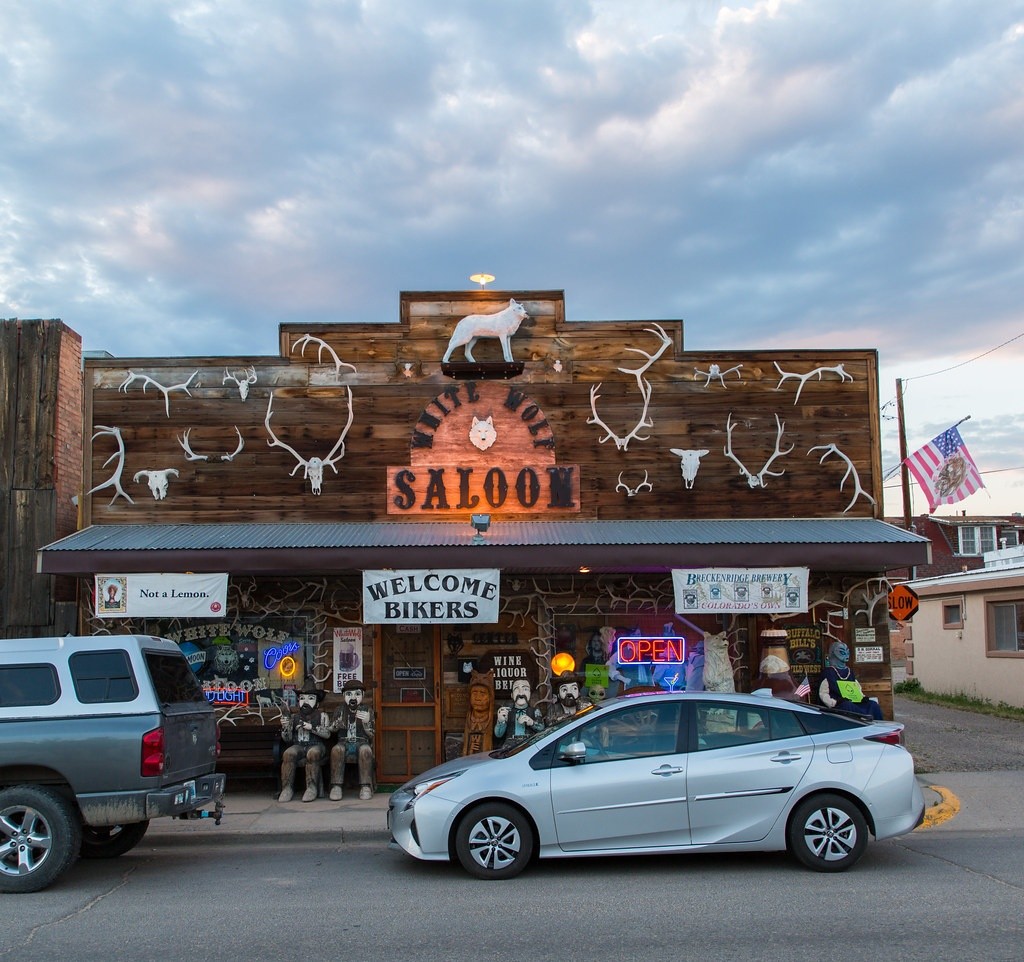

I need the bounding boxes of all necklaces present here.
[829,665,851,680]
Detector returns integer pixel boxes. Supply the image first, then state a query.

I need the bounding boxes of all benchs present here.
[215,725,373,799]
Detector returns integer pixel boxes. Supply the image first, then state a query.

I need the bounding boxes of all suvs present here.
[0,633,227,893]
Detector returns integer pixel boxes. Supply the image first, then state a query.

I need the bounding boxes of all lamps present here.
[469,273,495,289]
[470,514,491,541]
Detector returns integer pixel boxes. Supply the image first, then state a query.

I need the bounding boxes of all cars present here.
[386,690,925,881]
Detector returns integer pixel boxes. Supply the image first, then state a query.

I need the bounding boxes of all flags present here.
[903,427,984,513]
[794,678,810,697]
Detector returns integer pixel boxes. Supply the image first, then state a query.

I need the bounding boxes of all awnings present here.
[36,520,930,572]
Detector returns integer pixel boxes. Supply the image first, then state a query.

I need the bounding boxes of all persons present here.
[494,670,588,751]
[819,641,884,721]
[278,680,376,803]
[462,685,493,757]
